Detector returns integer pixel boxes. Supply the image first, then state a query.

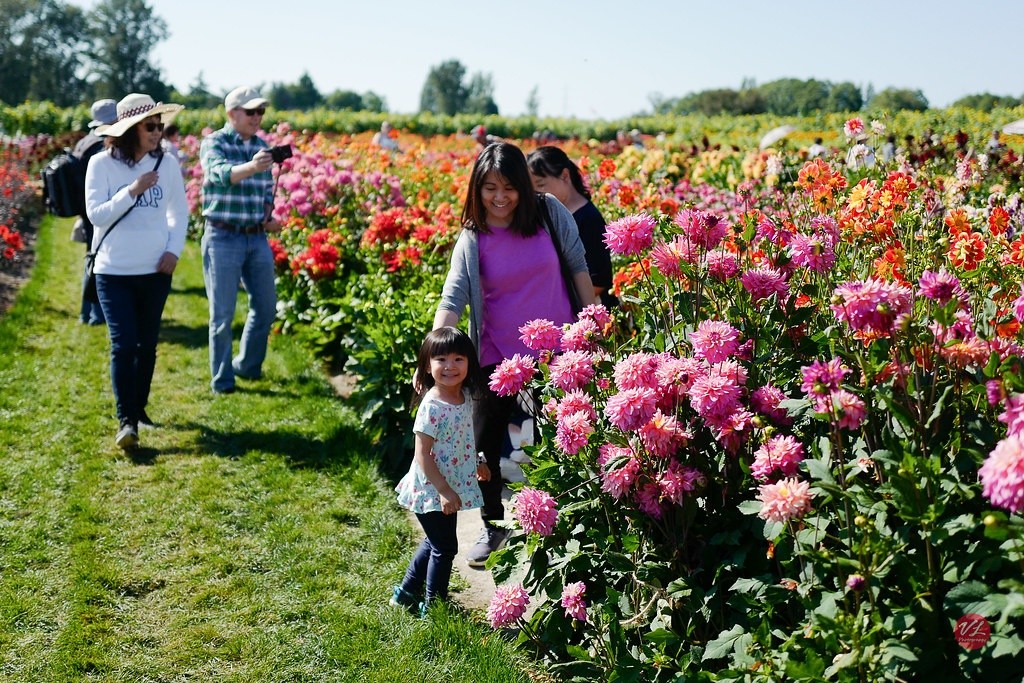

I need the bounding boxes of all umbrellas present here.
[759,125,799,151]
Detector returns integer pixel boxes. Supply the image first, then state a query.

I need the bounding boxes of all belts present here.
[207,221,263,233]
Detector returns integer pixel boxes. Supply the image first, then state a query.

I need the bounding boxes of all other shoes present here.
[116,425,139,452]
[137,409,156,427]
[389,587,422,614]
[419,602,428,619]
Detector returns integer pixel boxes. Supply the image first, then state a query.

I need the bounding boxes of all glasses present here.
[233,107,265,116]
[137,122,165,133]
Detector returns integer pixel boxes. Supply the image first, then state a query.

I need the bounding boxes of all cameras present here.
[264,144,293,164]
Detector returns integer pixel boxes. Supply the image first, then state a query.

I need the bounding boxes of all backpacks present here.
[39,137,104,217]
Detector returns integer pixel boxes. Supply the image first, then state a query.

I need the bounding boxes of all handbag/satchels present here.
[82,252,99,304]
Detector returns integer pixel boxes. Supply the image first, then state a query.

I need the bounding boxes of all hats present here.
[88,98,119,128]
[225,86,269,112]
[94,93,184,137]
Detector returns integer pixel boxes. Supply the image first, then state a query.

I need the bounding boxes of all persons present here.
[389,326,491,620]
[371,121,399,149]
[808,128,1003,171]
[412,142,596,568]
[455,125,667,156]
[72,97,118,326]
[199,86,277,394]
[525,145,620,314]
[159,124,188,179]
[84,93,189,450]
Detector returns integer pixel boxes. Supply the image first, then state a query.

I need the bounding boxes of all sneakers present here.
[466,526,513,567]
